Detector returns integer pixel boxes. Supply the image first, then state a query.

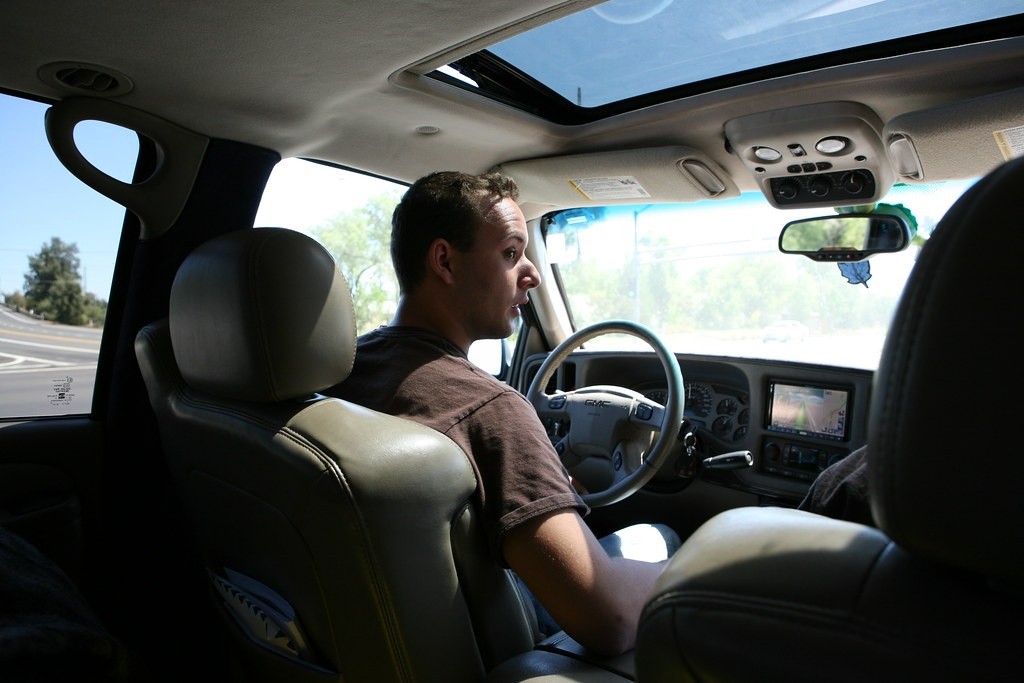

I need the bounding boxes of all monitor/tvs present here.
[765,379,853,442]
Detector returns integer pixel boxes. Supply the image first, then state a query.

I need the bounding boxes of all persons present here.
[313,168,683,660]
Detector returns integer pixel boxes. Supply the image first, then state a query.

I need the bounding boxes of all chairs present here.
[133,225,540,683]
[635,152,1023,683]
